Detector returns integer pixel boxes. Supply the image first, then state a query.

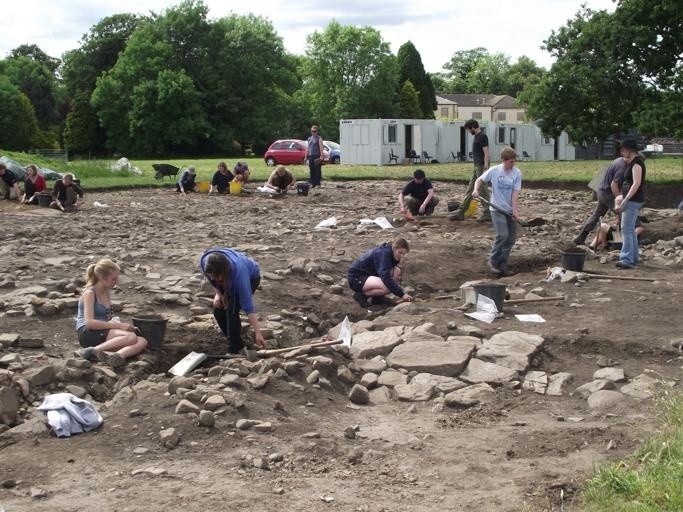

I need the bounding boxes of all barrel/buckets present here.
[448,202,459,212]
[132,315,168,348]
[229,182,242,194]
[37,193,52,206]
[199,182,209,193]
[608,241,623,251]
[297,184,309,196]
[473,283,506,312]
[560,249,587,272]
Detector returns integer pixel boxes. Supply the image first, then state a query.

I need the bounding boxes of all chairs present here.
[522,151,532,161]
[391,148,399,158]
[388,153,397,165]
[410,150,421,164]
[447,152,473,163]
[422,151,433,164]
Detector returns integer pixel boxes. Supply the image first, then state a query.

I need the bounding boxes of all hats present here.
[189,166,196,174]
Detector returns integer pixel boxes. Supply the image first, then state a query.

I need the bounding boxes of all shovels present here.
[612,208,623,242]
[637,215,649,223]
[477,195,548,226]
[168,350,247,377]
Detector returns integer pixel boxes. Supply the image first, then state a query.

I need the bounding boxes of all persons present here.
[208,163,238,194]
[471,147,521,275]
[199,248,266,354]
[234,161,249,185]
[76,259,147,369]
[0,164,83,211]
[449,120,491,222]
[573,138,645,268]
[303,125,323,189]
[175,165,198,193]
[399,170,439,216]
[347,238,413,308]
[266,165,295,195]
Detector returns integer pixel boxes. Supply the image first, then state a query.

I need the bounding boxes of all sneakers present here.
[371,296,389,304]
[487,261,501,274]
[494,269,514,279]
[352,292,368,308]
[448,210,464,220]
[477,210,491,222]
[220,353,242,365]
[426,210,433,216]
[100,351,125,367]
[239,346,249,360]
[411,210,419,216]
[74,347,99,362]
[615,261,631,268]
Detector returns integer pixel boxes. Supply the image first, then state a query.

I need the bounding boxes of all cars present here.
[261,136,343,167]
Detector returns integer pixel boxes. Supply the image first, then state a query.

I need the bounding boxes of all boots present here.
[573,231,589,246]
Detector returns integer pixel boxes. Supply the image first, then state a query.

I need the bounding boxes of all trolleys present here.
[152,160,187,182]
